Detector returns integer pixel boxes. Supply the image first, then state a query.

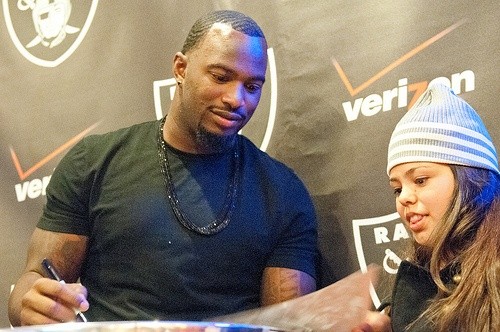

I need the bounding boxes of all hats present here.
[386,84,500,176]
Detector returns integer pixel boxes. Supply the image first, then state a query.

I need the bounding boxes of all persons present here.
[7,9,390,332]
[376,79,500,332]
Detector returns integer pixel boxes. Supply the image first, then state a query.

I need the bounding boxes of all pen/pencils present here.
[42,258,88,322]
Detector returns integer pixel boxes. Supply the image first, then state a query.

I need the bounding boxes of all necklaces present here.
[157,113,240,235]
